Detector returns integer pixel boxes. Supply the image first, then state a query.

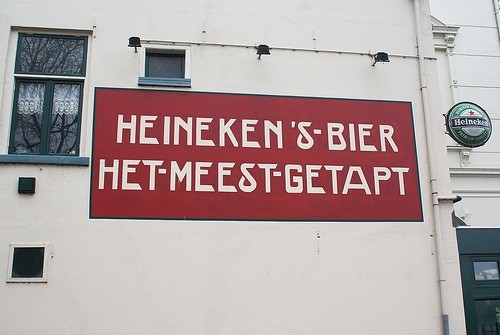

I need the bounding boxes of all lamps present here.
[128,37,141,51]
[372,52,390,65]
[256,45,271,59]
